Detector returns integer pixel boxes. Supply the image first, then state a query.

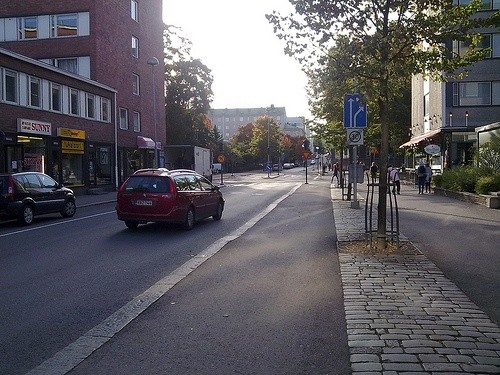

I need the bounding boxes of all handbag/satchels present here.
[418,173,423,177]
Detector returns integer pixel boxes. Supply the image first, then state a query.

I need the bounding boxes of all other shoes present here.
[428,190,431,194]
[420,193,421,194]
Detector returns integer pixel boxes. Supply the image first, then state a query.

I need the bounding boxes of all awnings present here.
[399,130,438,147]
[137,137,155,149]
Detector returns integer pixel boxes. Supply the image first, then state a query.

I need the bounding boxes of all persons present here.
[370,162,378,182]
[390,168,401,195]
[330,164,339,185]
[423,164,432,194]
[416,162,427,195]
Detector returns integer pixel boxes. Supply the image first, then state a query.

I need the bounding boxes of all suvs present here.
[116,168,225,231]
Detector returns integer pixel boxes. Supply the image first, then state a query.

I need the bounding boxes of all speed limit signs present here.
[346,129,364,145]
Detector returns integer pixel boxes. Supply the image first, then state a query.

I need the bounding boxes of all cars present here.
[273,162,295,171]
[0,171,77,226]
